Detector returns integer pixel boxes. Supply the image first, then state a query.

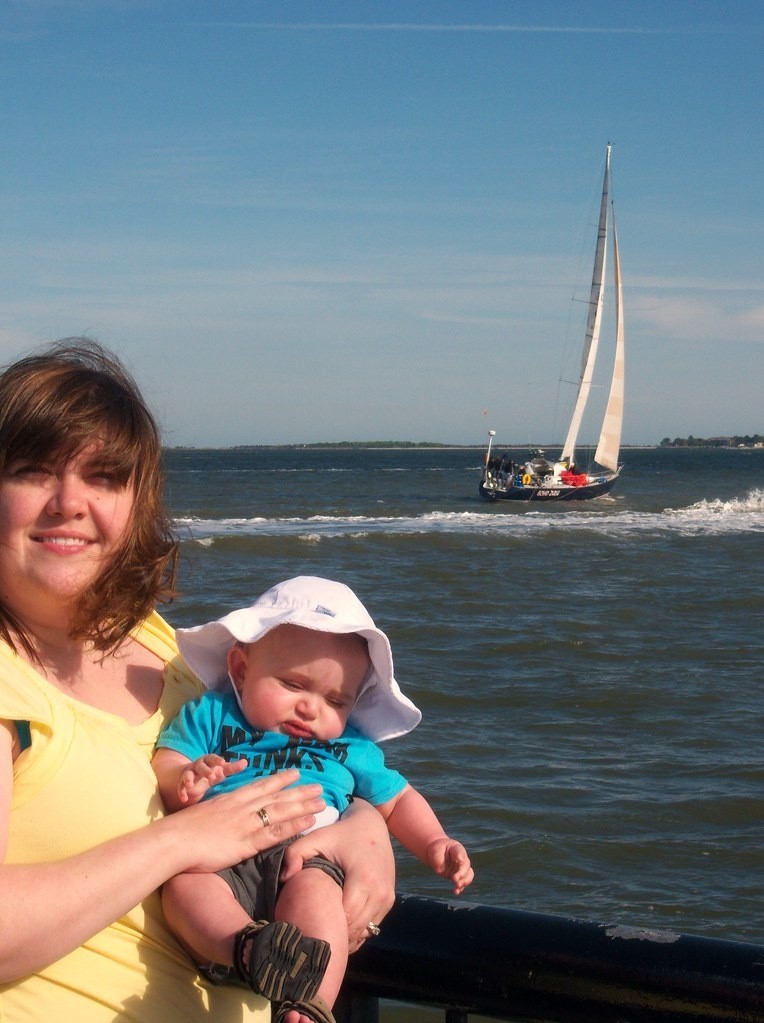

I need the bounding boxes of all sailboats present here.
[477,143,631,501]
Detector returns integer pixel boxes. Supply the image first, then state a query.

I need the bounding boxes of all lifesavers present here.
[522,474,531,485]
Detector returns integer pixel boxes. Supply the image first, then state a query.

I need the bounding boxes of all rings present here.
[367,921,381,936]
[257,806,271,827]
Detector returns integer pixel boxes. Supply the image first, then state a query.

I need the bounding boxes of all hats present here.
[175,575,422,744]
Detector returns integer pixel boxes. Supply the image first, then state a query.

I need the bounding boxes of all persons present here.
[483,444,585,494]
[154,576,473,1022]
[2,336,396,1022]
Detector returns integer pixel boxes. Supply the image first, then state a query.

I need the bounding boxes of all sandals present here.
[272,994,338,1023]
[234,919,331,1006]
